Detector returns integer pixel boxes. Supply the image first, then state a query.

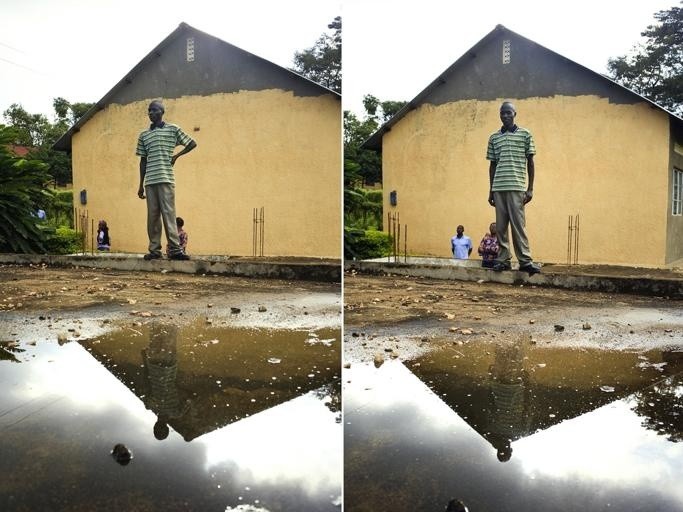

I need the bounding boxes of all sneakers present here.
[493,264,512,271]
[144,253,164,260]
[167,253,191,261]
[519,265,541,274]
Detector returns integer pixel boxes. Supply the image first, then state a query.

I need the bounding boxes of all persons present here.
[488,344,533,462]
[135,100,197,267]
[97,220,110,252]
[141,324,200,440]
[30,202,46,220]
[450,223,500,260]
[485,102,536,273]
